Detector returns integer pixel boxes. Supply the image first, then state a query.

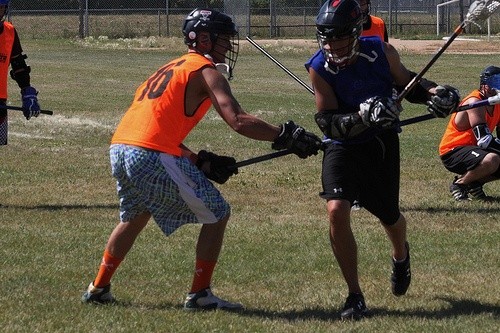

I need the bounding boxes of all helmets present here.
[0,0,9,24]
[314,0,363,77]
[354,0,372,24]
[182,7,240,80]
[479,66,500,98]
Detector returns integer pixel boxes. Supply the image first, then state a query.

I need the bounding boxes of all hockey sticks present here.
[0,104,54,116]
[398,0,500,100]
[245,35,315,96]
[236,90,500,167]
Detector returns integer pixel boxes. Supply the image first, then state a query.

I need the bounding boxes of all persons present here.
[81,7,328,313]
[438,65,500,203]
[0,0,41,145]
[306,0,461,321]
[355,0,388,43]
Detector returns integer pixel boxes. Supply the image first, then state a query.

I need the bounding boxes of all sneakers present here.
[449,176,469,202]
[184,288,242,313]
[390,241,412,296]
[468,186,486,201]
[340,293,368,318]
[81,280,118,307]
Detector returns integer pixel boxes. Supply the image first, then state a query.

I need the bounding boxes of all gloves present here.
[427,84,461,118]
[358,94,403,131]
[20,86,40,120]
[271,120,326,159]
[196,151,239,184]
